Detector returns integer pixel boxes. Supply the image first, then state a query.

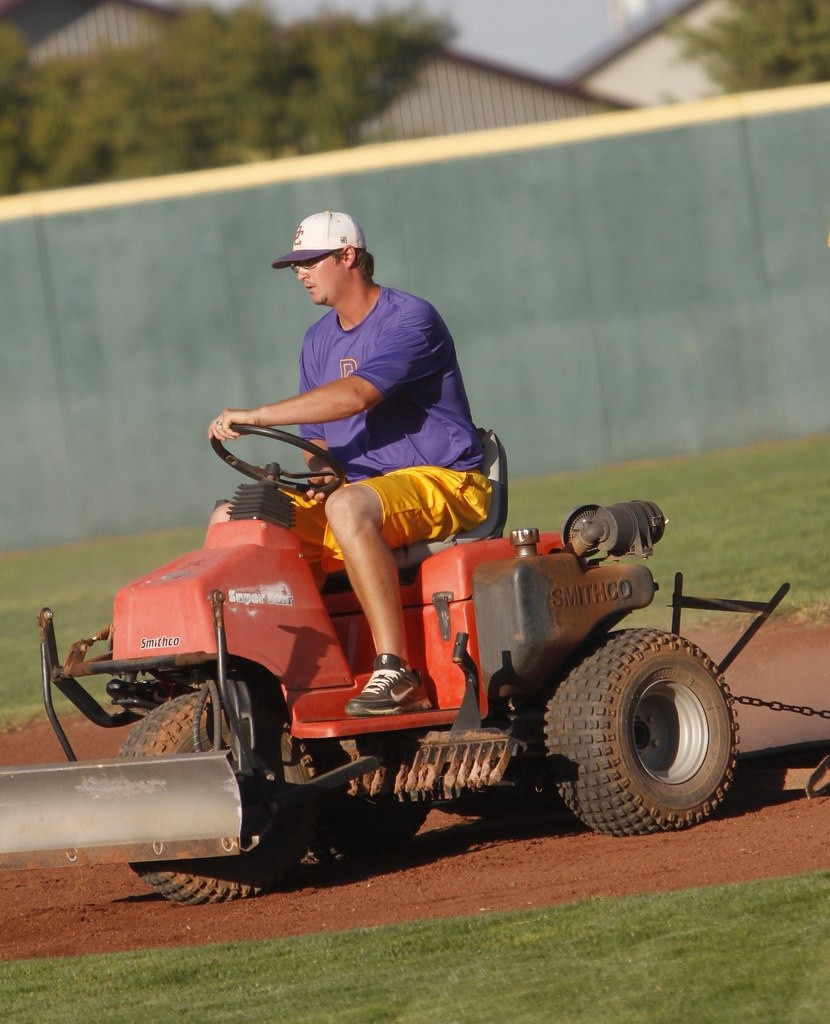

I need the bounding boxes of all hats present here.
[271,210,367,269]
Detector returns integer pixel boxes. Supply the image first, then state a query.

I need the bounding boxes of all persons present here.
[207,210,491,719]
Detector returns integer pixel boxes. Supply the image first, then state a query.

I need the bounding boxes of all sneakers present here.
[345,653,432,715]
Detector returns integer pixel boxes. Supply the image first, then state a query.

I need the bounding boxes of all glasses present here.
[290,251,339,273]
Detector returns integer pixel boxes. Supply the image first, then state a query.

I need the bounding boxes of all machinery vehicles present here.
[0,424,830,908]
